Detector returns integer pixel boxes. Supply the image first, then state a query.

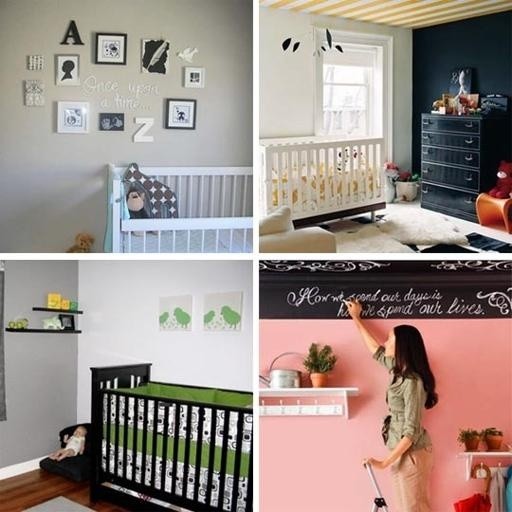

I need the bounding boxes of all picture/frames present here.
[55,33,206,133]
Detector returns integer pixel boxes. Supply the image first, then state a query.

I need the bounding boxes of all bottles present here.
[452,103,481,116]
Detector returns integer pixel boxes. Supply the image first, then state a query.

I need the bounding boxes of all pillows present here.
[259,206,295,235]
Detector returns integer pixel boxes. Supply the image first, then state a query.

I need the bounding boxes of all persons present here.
[346,301,438,512]
[454,71,471,100]
[47,426,87,462]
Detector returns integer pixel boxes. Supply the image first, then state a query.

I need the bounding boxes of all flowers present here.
[385,161,397,178]
[394,171,419,182]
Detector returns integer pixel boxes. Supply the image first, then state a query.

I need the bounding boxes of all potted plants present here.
[481,427,504,450]
[303,343,337,387]
[457,430,481,450]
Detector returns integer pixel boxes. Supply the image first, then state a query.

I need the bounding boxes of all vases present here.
[386,176,395,203]
[395,182,418,201]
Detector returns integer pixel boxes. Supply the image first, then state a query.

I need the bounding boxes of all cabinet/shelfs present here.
[421,112,511,223]
[59,314,74,330]
[5,307,83,333]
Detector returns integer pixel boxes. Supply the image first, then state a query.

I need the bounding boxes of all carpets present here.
[316,212,512,254]
[22,496,97,512]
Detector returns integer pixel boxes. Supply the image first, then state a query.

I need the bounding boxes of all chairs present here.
[40,423,92,483]
[259,226,336,253]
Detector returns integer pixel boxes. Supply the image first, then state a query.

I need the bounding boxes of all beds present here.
[89,362,253,512]
[259,135,386,230]
[106,163,253,253]
